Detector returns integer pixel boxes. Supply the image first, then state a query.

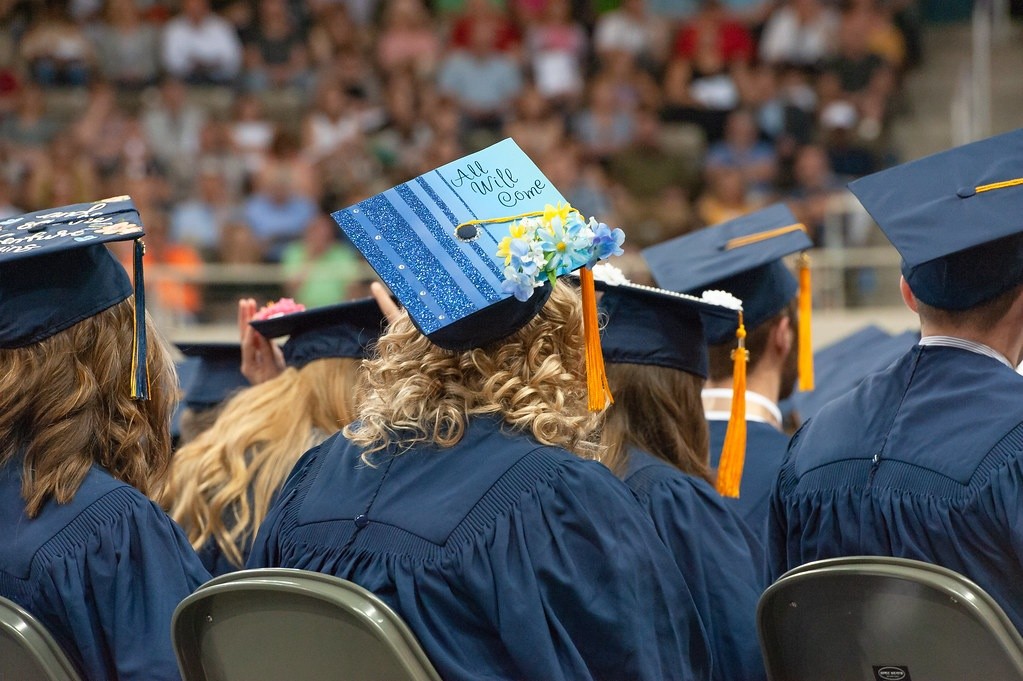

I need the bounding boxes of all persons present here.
[0,0,928,681]
[769,134,1021,635]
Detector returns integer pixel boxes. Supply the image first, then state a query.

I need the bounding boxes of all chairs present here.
[756,556,1023,681]
[0,595,82,681]
[170,568,442,681]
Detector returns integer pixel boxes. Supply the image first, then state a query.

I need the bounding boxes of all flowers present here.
[496,205,627,301]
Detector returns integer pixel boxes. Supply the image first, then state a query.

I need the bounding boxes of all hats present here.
[564,261,749,498]
[641,200,815,390]
[0,195,152,402]
[173,337,254,411]
[246,291,405,370]
[330,137,626,410]
[849,125,1023,312]
